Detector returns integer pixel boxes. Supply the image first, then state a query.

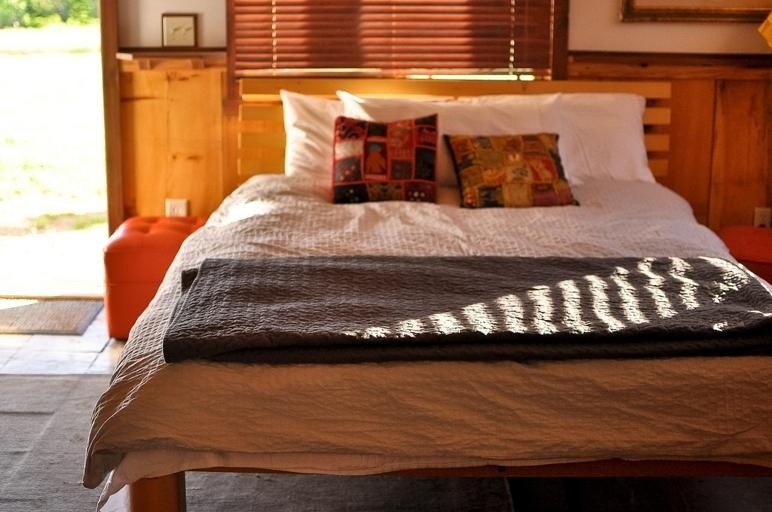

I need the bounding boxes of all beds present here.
[82,76,772,512]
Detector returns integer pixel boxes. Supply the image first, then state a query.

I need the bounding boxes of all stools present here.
[103,216,208,341]
[720,226,772,286]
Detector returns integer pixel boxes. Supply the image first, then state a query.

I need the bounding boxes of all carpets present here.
[0,373,772,512]
[0,297,105,335]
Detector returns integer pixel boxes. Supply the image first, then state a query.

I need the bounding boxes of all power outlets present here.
[753,207,772,228]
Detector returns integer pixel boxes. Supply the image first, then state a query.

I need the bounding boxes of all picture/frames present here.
[619,0,772,23]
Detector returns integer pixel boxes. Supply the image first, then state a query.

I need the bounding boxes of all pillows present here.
[458,92,654,183]
[332,112,440,205]
[281,87,453,178]
[335,87,573,180]
[444,131,580,208]
